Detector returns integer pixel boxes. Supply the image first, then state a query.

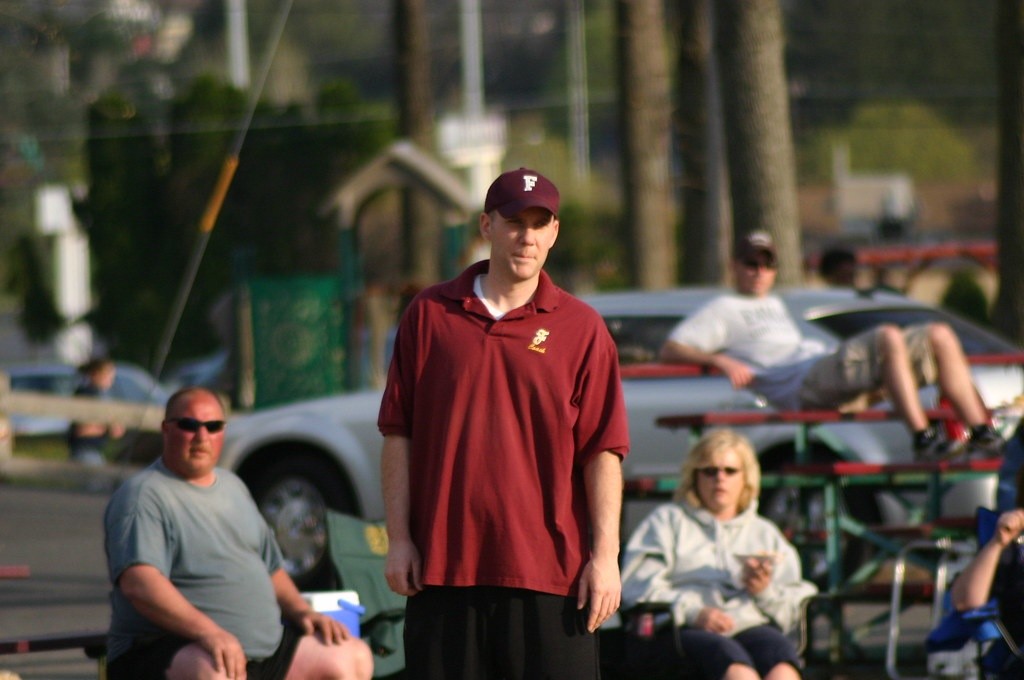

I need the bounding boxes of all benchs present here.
[621,353,1024,680]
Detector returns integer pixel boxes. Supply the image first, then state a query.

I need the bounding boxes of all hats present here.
[736,229,776,260]
[484,166,559,222]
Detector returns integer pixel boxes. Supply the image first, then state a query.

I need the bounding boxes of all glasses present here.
[697,466,745,476]
[741,257,780,270]
[164,417,226,434]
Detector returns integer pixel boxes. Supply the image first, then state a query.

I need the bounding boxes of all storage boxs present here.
[301,590,367,638]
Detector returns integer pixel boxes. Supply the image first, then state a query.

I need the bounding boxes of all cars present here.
[2,354,184,444]
[209,279,1023,615]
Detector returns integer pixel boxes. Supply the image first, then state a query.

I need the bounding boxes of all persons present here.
[380,171,627,680]
[109,231,1024,680]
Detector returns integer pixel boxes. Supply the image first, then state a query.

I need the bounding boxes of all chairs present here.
[323,508,408,680]
[619,593,845,680]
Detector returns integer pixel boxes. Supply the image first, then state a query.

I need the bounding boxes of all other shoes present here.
[915,430,962,462]
[968,422,1016,452]
